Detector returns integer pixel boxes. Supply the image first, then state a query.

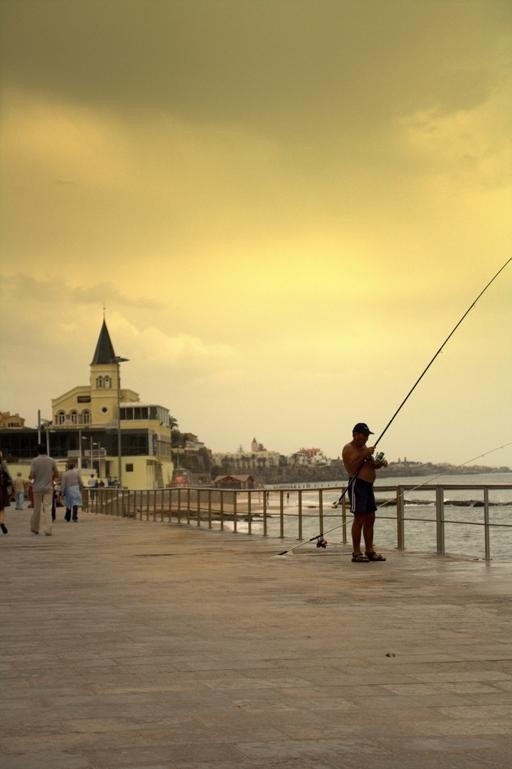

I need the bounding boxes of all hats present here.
[353,423,373,433]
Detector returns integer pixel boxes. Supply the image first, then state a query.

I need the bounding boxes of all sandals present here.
[352,551,385,561]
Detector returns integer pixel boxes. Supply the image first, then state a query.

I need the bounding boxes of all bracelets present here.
[358,455,364,461]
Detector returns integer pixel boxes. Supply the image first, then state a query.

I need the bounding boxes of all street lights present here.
[81,435,93,470]
[177,445,182,468]
[115,357,130,488]
[45,425,56,457]
[92,440,101,477]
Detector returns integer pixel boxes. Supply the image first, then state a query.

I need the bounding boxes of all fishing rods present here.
[332,256,512,508]
[275,444,512,556]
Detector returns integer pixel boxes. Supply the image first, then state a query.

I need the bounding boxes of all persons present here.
[342,422,388,563]
[0,444,120,536]
